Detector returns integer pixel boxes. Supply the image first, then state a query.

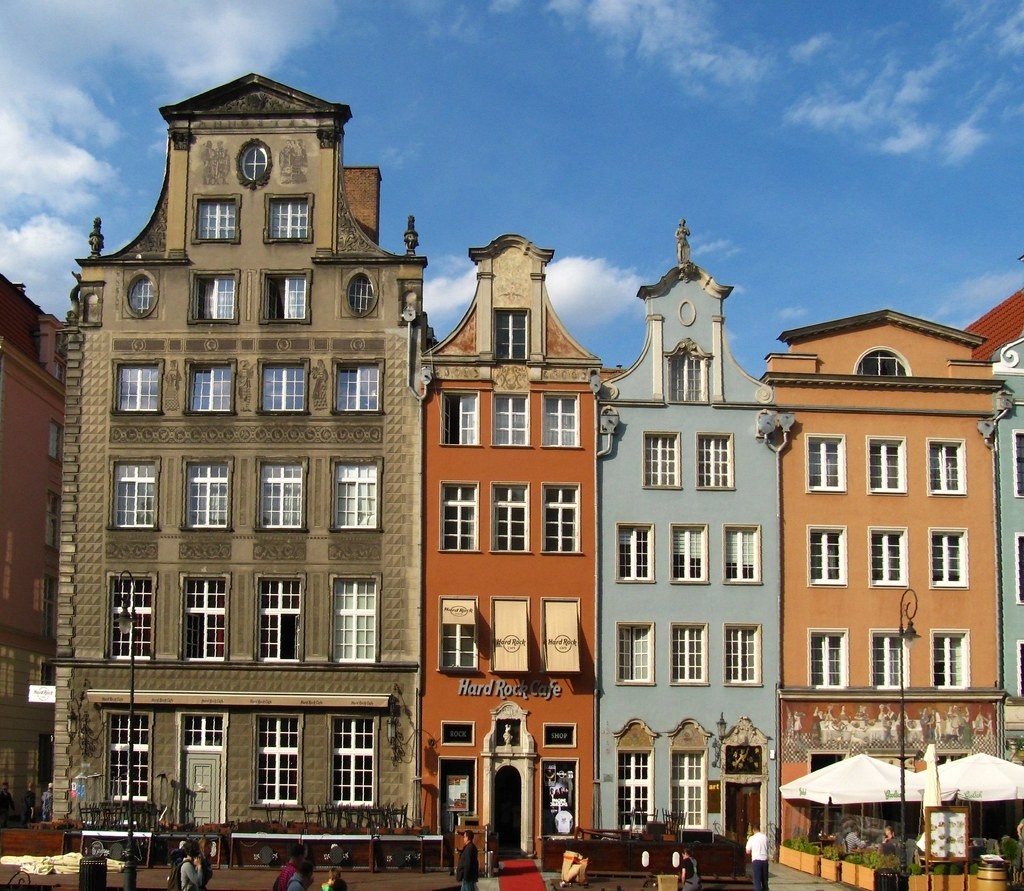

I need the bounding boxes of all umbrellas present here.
[778,753,923,839]
[917,752,1024,846]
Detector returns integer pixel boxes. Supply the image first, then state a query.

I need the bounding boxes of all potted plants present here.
[779,837,979,891]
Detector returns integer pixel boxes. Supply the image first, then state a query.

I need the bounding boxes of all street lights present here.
[899,588,921,891]
[113,569,136,891]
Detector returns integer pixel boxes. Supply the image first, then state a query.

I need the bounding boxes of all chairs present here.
[38,803,421,834]
[618,809,688,839]
[976,836,1024,881]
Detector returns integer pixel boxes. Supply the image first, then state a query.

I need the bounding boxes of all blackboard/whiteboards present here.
[926,805,971,863]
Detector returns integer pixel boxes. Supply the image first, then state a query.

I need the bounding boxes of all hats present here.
[48,783,53,788]
[178,841,187,849]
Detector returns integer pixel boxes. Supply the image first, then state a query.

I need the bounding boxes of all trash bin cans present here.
[78,856,108,891]
[873,866,898,890]
[976,853,1008,891]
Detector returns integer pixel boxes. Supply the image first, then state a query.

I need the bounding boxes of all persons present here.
[880,824,896,843]
[38,782,53,821]
[168,836,214,891]
[273,844,312,891]
[679,848,702,891]
[745,823,770,891]
[674,219,690,264]
[845,825,867,849]
[456,830,479,891]
[0,782,15,828]
[1016,819,1024,839]
[21,782,36,829]
[321,868,347,891]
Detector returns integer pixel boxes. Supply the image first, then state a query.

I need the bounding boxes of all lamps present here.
[712,712,728,768]
[384,701,406,764]
[64,697,91,758]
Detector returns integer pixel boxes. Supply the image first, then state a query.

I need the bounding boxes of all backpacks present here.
[168,860,195,891]
